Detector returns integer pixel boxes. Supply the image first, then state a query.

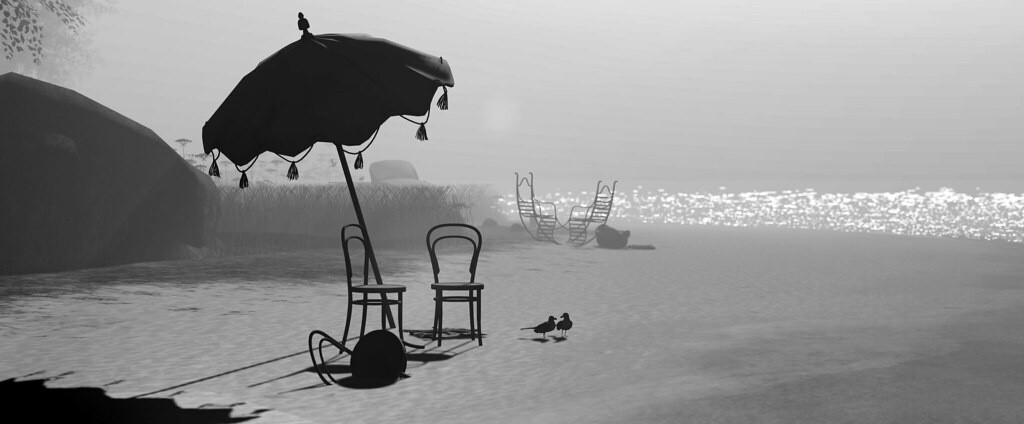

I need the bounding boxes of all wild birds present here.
[520,312,573,339]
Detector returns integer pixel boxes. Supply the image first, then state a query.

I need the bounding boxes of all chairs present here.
[426,223,485,347]
[338,223,407,360]
[515,172,618,247]
[308,329,411,388]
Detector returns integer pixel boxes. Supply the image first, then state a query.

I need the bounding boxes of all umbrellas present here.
[201,12,455,329]
